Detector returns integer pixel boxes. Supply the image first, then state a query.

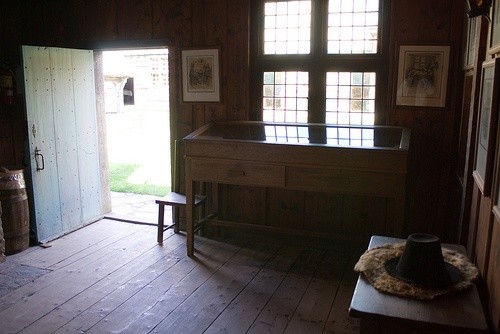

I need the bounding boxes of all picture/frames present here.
[472,57,500,197]
[178,47,224,103]
[392,41,453,111]
[463,11,484,70]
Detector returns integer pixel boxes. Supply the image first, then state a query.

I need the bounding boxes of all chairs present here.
[155,139,205,243]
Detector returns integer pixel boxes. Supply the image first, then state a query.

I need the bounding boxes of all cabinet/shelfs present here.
[183,120,412,257]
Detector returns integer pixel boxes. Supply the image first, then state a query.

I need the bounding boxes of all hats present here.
[353,232,480,302]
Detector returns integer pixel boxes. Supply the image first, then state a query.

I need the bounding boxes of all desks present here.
[348,235,488,334]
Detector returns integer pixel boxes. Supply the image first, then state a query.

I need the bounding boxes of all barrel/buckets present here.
[0,168,30,256]
